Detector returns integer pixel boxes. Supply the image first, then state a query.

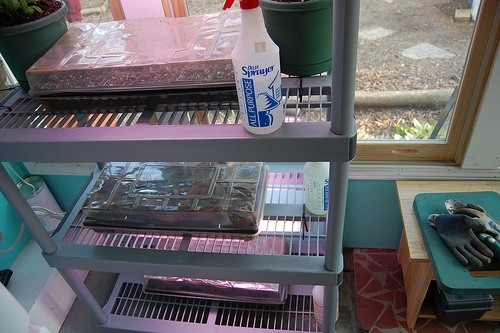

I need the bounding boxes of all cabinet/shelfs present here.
[0,0,360,332]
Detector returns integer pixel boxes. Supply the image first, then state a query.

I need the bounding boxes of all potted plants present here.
[0,0,68,90]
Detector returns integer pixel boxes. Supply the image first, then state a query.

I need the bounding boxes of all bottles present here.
[222,0,284,134]
[313,285,339,324]
[304,162,330,215]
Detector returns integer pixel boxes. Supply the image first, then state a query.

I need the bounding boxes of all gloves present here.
[428,212,493,269]
[446,198,500,267]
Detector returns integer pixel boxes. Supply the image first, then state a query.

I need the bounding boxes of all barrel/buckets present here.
[16,176,65,236]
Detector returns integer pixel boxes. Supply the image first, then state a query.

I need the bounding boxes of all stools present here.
[396,180,500,329]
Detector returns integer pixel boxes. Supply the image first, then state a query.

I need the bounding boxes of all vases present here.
[261,0,333,76]
